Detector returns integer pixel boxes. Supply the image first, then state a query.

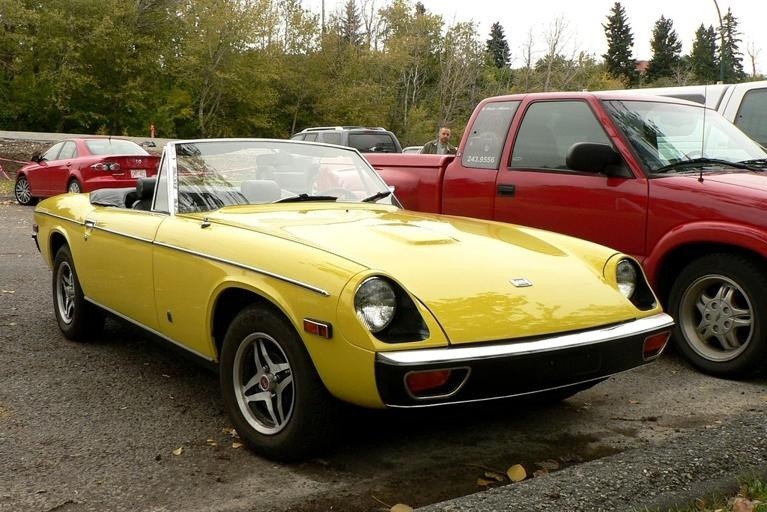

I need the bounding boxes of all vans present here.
[256,124,404,193]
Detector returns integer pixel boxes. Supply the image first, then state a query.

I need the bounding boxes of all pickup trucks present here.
[362,90,766,380]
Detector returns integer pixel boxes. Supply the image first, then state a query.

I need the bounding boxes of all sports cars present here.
[30,139,678,464]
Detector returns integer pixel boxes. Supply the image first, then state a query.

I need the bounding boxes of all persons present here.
[420,127,457,154]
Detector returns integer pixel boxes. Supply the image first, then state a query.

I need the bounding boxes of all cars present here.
[13,138,162,207]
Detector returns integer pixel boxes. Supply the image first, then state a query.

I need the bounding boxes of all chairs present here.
[133,172,283,210]
